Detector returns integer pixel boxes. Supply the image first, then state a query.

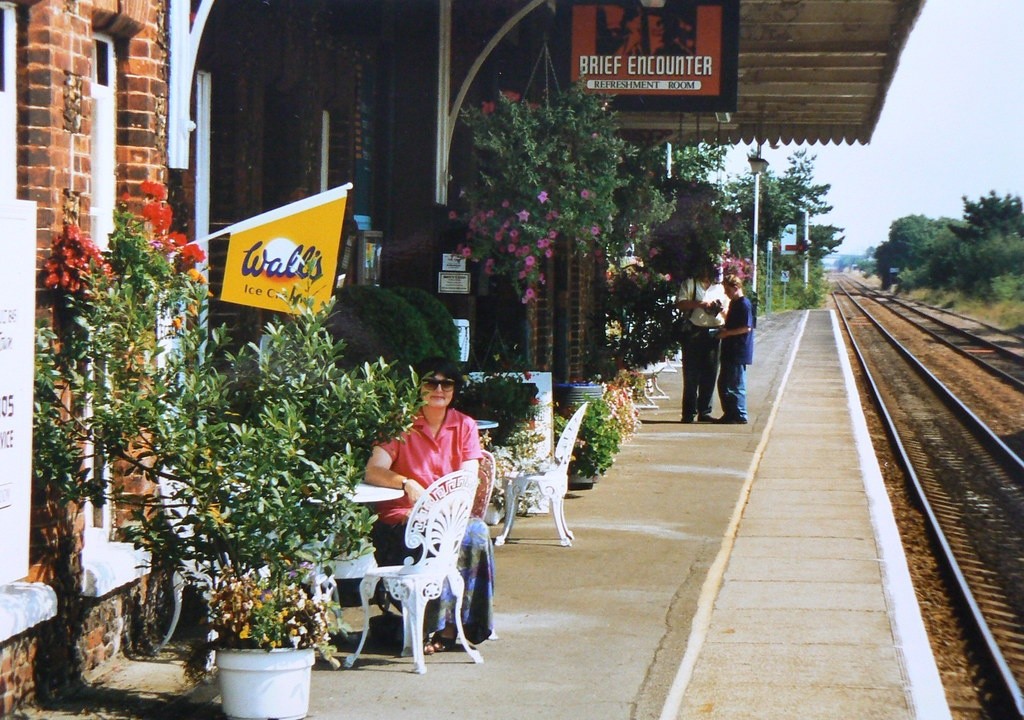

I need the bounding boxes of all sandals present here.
[432,622,457,650]
[421,628,434,654]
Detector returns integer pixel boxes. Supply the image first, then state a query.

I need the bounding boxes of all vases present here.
[567,460,596,491]
[216,649,316,720]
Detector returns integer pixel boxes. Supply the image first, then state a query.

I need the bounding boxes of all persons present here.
[364,356,495,657]
[677,261,730,423]
[712,274,756,423]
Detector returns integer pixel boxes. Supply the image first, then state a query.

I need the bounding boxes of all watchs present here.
[401,477,410,490]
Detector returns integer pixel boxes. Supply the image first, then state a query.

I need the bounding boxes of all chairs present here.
[494,401,589,546]
[157,465,268,673]
[470,449,498,519]
[348,470,485,675]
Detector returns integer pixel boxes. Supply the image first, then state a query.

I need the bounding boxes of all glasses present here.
[420,377,456,391]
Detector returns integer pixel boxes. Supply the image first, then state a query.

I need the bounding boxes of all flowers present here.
[109,182,214,333]
[204,559,343,672]
[41,219,116,304]
[583,370,652,479]
[475,428,549,517]
[449,85,679,305]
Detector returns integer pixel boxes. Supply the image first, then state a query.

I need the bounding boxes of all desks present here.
[238,475,405,668]
[472,421,499,433]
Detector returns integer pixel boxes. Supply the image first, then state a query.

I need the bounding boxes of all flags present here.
[220,187,347,317]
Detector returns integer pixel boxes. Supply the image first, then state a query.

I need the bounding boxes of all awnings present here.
[573,0,929,147]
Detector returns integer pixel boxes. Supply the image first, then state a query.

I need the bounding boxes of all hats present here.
[708,260,724,284]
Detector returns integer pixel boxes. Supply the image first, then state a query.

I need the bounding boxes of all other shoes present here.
[680,414,693,422]
[697,413,718,422]
[716,414,748,423]
